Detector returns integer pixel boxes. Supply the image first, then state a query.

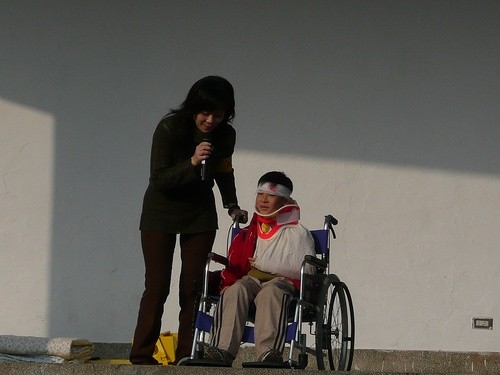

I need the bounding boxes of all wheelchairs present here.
[177,212,354,371]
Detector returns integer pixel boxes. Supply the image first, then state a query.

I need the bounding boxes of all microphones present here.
[201,132,212,180]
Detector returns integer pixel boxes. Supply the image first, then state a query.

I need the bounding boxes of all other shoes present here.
[204,347,234,367]
[259,349,284,363]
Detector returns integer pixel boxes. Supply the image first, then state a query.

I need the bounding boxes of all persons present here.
[204,170,316,362]
[128,75,249,366]
